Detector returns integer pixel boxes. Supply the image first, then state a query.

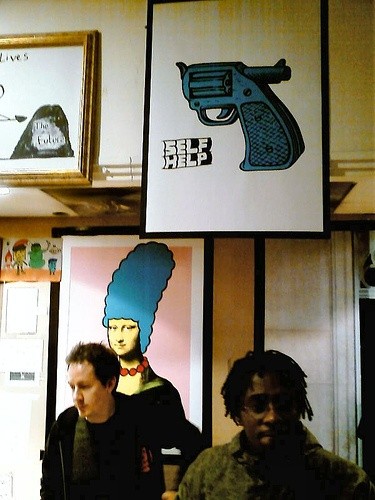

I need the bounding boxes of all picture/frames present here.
[0,30,100,188]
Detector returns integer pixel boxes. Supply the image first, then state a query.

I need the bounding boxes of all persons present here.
[177,350,375,500]
[39,342,164,500]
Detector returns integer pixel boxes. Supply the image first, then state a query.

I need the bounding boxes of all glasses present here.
[241,399,295,413]
